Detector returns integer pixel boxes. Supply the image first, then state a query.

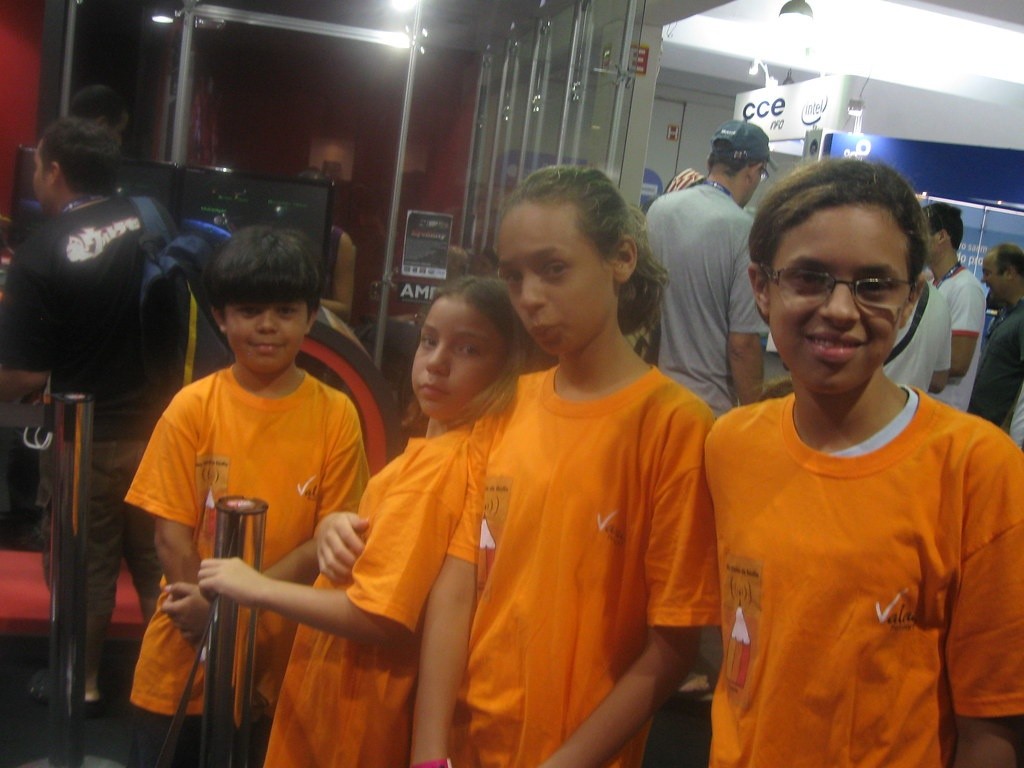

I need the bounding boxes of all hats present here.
[711,120,778,172]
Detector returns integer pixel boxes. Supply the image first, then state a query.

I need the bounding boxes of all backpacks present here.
[125,190,238,411]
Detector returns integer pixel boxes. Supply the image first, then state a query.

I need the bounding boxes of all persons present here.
[880,282,953,404]
[639,122,770,706]
[0,86,356,768]
[923,200,987,413]
[124,224,373,768]
[967,239,1024,454]
[196,276,534,768]
[702,159,1024,768]
[409,167,721,768]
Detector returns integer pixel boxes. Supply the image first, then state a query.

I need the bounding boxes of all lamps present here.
[848,99,863,134]
[748,58,794,88]
[779,0,813,20]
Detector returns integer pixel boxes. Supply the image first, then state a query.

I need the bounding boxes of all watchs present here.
[410,757,452,768]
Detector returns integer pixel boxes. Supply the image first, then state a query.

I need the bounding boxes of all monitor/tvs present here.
[11,145,336,299]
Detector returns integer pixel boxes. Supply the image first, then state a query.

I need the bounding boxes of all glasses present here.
[757,163,769,182]
[761,262,917,310]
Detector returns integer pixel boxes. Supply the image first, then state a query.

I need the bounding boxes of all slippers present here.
[29,668,107,718]
